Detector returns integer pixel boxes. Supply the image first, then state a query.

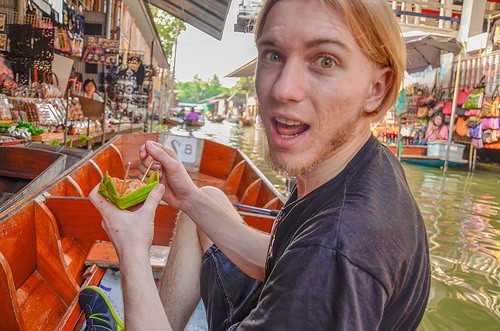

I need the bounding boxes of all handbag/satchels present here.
[42,68,62,98]
[48,1,86,57]
[416,75,500,152]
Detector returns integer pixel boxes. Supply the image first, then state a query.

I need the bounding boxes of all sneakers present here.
[78,286,124,331]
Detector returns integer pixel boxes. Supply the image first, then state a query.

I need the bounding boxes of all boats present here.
[0,142,94,219]
[164,111,254,127]
[395,154,469,168]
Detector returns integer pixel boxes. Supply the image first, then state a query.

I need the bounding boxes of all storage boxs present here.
[427,141,466,162]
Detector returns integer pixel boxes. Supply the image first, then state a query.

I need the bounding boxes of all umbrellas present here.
[395,29,464,160]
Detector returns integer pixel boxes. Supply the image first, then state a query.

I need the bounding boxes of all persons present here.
[88,0,432,331]
[424,111,449,143]
[79,78,104,103]
[177,104,203,122]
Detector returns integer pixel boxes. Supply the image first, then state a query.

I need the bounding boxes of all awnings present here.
[147,0,233,41]
